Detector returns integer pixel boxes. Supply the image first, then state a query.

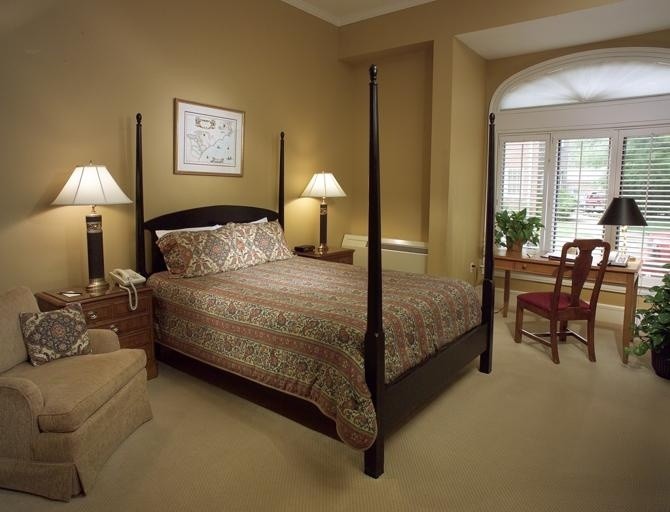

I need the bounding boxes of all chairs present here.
[1,285,155,504]
[514,240,611,364]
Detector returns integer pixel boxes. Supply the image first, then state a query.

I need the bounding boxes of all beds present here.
[134,62,498,479]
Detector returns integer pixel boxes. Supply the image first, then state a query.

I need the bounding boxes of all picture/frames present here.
[172,97,246,177]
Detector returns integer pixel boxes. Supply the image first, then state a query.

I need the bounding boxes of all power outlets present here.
[469,262,476,272]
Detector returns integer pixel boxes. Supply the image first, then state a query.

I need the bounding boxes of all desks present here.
[492,251,644,365]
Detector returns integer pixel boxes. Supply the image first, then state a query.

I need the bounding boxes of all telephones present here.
[109,268,147,288]
[597,250,630,267]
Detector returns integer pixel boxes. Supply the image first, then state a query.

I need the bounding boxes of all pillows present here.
[155,222,221,273]
[155,226,242,278]
[227,218,294,272]
[18,302,92,367]
[247,217,268,224]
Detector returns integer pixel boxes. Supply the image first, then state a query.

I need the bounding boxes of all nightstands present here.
[293,248,356,265]
[33,285,159,381]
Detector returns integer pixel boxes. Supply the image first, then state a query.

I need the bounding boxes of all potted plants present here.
[623,263,670,380]
[493,208,546,252]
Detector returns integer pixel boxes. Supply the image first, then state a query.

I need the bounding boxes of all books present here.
[549,251,577,263]
[541,250,553,258]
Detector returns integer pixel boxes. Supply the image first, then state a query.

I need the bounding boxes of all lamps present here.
[50,160,135,292]
[298,169,347,252]
[596,196,649,254]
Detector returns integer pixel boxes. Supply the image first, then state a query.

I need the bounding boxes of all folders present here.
[548,250,576,262]
